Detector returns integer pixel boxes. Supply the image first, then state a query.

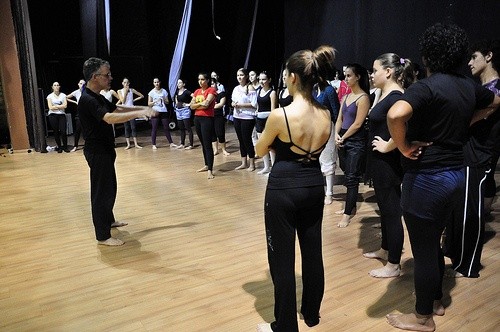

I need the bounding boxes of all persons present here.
[312,60,420,238]
[77,57,158,246]
[66,79,86,152]
[176,78,195,150]
[441,35,500,278]
[99,87,122,147]
[47,81,70,153]
[361,52,416,278]
[189,72,216,179]
[255,44,337,332]
[148,77,177,150]
[385,19,500,332]
[231,67,258,172]
[249,69,276,175]
[117,77,144,150]
[210,71,231,156]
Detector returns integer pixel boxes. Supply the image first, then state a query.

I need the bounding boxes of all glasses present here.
[95,72,111,78]
